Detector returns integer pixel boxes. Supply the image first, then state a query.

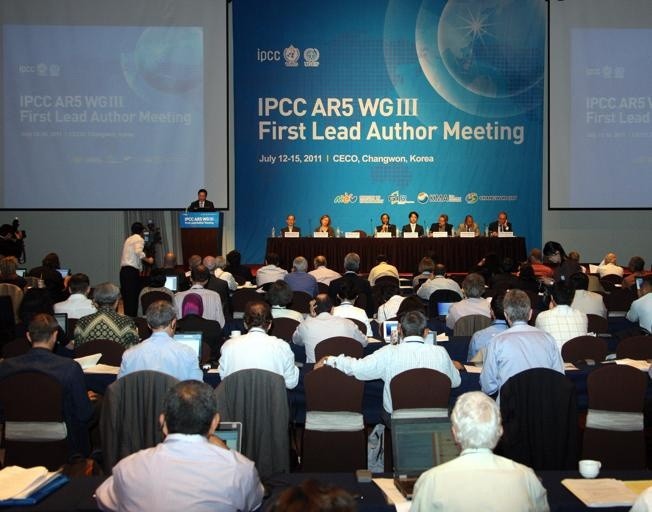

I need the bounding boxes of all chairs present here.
[0,264,651,510]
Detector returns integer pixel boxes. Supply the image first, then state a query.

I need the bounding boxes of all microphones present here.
[307,218,313,238]
[370,218,374,238]
[424,220,428,239]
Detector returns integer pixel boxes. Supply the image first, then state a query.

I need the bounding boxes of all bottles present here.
[484,224,489,237]
[336,226,340,238]
[426,226,430,237]
[272,227,275,238]
[451,227,455,237]
[373,227,377,238]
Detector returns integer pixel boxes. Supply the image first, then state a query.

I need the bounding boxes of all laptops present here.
[54,313,68,336]
[398,331,437,345]
[171,331,204,364]
[56,268,71,279]
[163,275,179,291]
[382,320,399,344]
[214,421,243,454]
[15,268,27,278]
[437,301,459,322]
[635,276,644,290]
[390,416,461,501]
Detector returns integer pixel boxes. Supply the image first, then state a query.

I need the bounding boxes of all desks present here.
[265,237,527,272]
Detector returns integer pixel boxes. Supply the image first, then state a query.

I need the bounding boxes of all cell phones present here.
[538,278,553,283]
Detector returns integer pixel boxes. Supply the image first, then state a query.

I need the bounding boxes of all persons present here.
[0,210,652,512]
[187,191,212,210]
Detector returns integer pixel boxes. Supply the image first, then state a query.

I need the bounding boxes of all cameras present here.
[10,216,27,240]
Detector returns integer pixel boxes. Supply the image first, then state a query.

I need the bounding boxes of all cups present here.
[246,281,251,286]
[230,330,241,338]
[579,459,602,478]
